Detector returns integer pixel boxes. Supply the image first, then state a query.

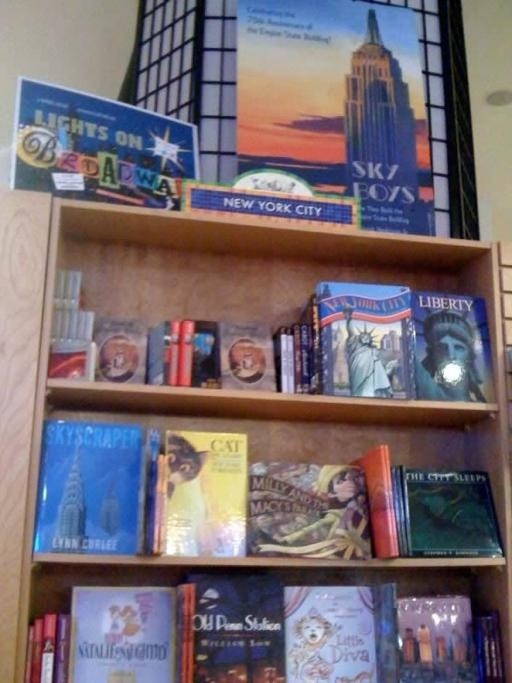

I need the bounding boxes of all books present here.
[249,463,372,556]
[394,593,503,682]
[280,583,401,681]
[153,429,248,556]
[405,468,502,556]
[148,320,222,390]
[218,320,277,392]
[46,270,97,380]
[34,419,159,556]
[315,282,494,401]
[92,317,149,384]
[175,576,286,681]
[25,609,71,681]
[68,583,196,681]
[349,445,413,556]
[272,293,320,394]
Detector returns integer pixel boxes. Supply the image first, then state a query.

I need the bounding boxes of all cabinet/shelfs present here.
[15,197,512,683]
